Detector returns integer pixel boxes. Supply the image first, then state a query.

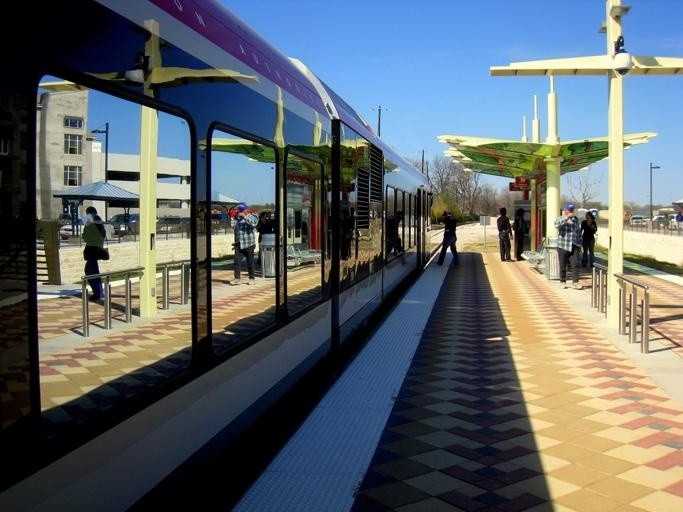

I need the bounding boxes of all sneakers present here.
[561,282,566,289]
[229,278,240,285]
[517,258,524,261]
[248,278,254,285]
[89,293,104,300]
[571,283,583,290]
[501,259,515,262]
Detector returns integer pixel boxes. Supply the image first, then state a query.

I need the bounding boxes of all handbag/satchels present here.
[84,246,108,260]
[498,230,509,240]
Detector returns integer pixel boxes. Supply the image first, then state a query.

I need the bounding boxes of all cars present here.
[60,213,190,240]
[212,213,231,230]
[630,214,683,231]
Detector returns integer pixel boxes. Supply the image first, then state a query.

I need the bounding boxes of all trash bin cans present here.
[259,234,276,278]
[543,238,561,280]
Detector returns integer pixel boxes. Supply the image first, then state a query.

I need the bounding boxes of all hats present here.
[564,202,575,210]
[238,204,247,209]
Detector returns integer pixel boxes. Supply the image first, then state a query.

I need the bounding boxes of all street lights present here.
[370,104,389,139]
[91,122,114,223]
[649,162,661,220]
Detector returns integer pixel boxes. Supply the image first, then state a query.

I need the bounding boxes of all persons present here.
[580,211,596,268]
[385,207,406,265]
[512,208,528,262]
[553,202,584,291]
[80,205,105,306]
[436,207,458,266]
[256,210,271,270]
[227,202,259,285]
[496,207,514,262]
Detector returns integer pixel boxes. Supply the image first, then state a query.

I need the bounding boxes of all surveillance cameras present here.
[613,53,631,76]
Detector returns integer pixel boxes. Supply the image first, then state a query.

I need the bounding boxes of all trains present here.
[0,0,435,512]
